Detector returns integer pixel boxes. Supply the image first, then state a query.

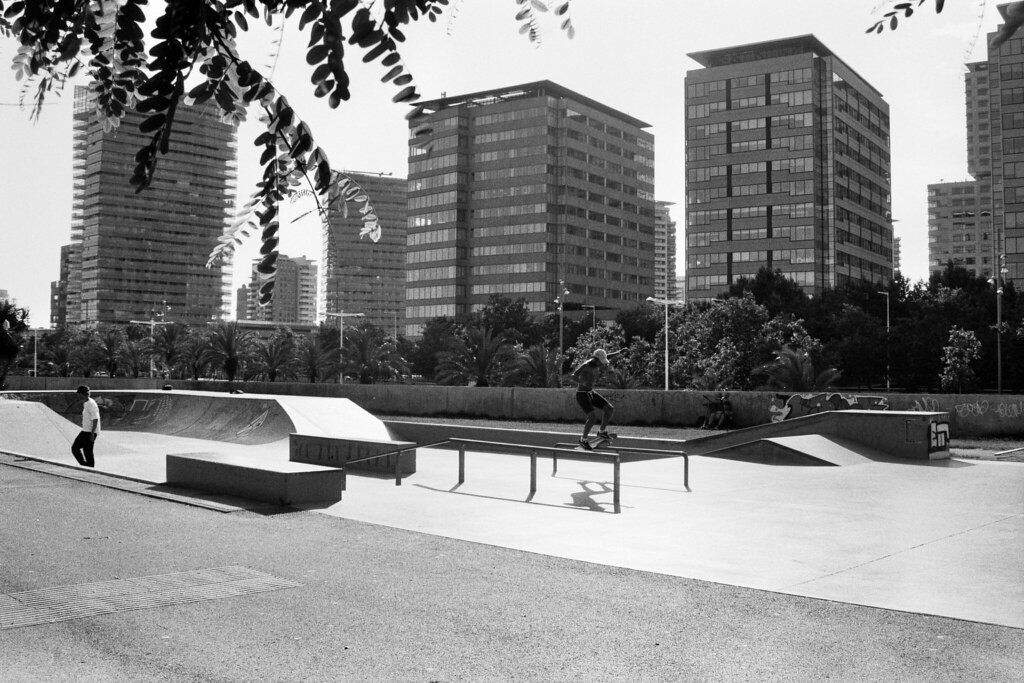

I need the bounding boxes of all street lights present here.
[878,289,892,391]
[582,302,596,335]
[986,252,1008,394]
[130,318,176,377]
[319,308,364,384]
[646,295,677,390]
[552,279,571,387]
[383,311,397,351]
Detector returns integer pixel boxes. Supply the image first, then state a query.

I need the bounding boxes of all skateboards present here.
[574,433,617,452]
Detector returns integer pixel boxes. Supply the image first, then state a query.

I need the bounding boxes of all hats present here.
[591,347,610,364]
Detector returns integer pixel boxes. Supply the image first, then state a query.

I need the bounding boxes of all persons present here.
[71,385,101,468]
[569,347,631,450]
[697,391,735,431]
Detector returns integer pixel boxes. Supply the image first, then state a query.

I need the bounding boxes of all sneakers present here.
[578,440,593,451]
[596,430,613,441]
